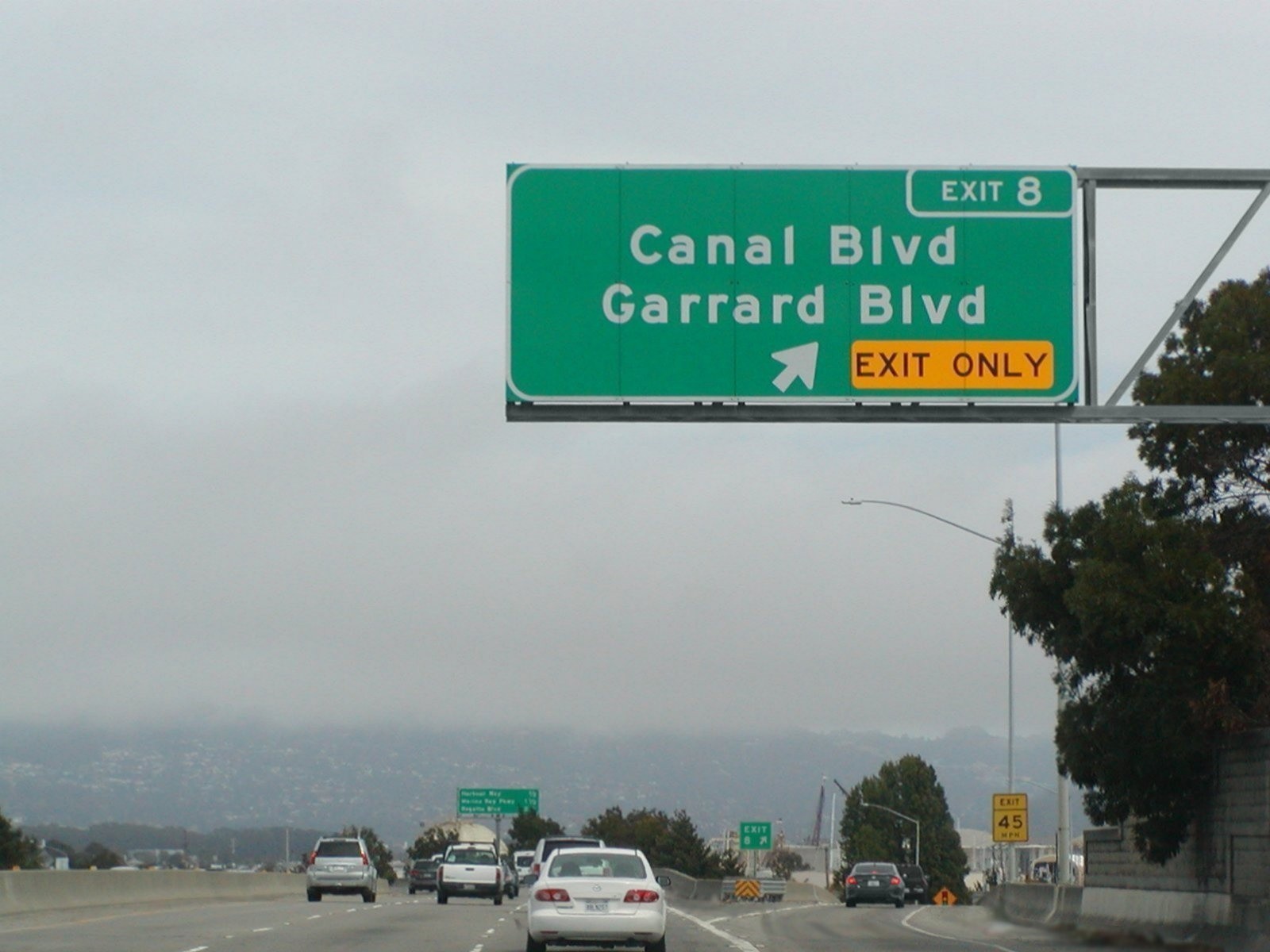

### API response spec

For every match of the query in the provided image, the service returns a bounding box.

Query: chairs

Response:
[480,855,490,864]
[614,863,637,877]
[560,863,581,876]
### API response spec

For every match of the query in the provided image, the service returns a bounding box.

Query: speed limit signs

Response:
[993,792,1030,843]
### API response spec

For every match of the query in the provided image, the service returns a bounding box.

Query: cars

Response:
[844,862,932,908]
[406,841,521,906]
[527,847,671,952]
[306,836,378,904]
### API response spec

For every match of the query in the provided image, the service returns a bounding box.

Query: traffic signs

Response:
[506,163,1087,407]
[738,821,771,850]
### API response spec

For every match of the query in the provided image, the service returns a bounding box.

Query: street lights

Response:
[859,800,921,866]
[844,496,1017,884]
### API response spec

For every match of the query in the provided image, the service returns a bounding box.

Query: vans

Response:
[512,837,616,882]
